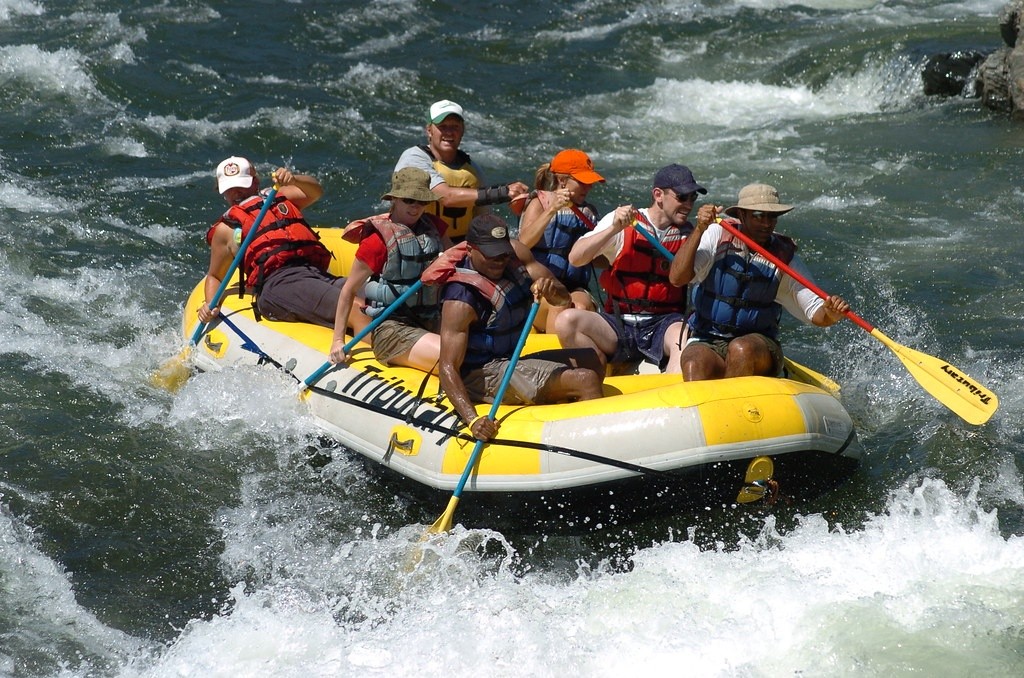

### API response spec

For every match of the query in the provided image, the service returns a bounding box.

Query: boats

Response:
[181,224,865,541]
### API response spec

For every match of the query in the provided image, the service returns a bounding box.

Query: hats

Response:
[217,156,256,195]
[466,213,514,258]
[655,164,707,195]
[724,180,794,218]
[380,167,443,201]
[426,99,465,125]
[549,149,606,185]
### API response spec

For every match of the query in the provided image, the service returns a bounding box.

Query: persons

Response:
[669,183,851,382]
[197,155,374,345]
[511,149,605,333]
[554,164,722,373]
[327,167,457,376]
[438,215,603,442]
[392,99,529,243]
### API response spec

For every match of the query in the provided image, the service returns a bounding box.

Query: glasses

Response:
[668,192,698,202]
[752,211,778,219]
[402,198,430,206]
[485,254,512,259]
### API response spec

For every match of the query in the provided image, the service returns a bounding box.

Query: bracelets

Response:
[468,416,481,430]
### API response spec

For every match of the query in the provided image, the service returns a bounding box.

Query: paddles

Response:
[629,219,841,395]
[567,201,595,230]
[298,251,444,394]
[401,293,544,571]
[148,172,281,395]
[717,215,999,426]
